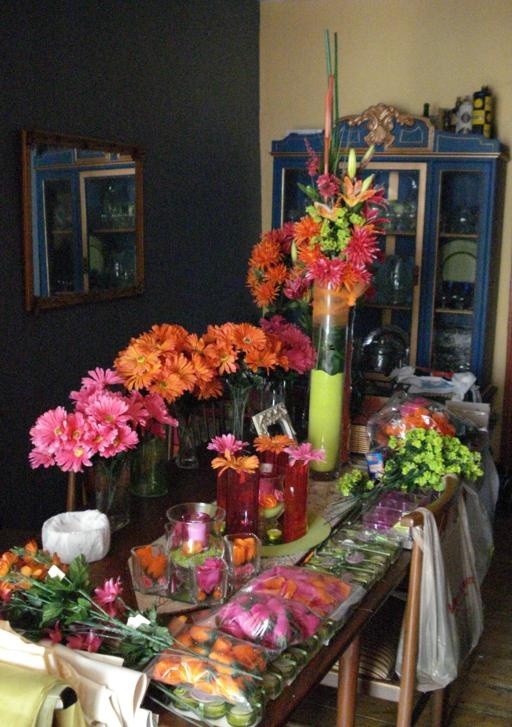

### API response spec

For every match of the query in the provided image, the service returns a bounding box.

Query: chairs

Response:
[320,478,463,724]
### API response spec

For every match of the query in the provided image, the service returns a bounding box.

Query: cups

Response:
[132,542,172,596]
[223,533,260,583]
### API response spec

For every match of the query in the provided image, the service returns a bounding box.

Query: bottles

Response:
[164,502,227,604]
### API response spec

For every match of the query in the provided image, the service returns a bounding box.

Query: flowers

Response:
[28,313,329,577]
[247,133,395,374]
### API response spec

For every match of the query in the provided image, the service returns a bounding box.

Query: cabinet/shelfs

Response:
[272,104,509,395]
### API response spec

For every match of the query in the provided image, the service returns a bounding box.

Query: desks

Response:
[91,416,438,727]
